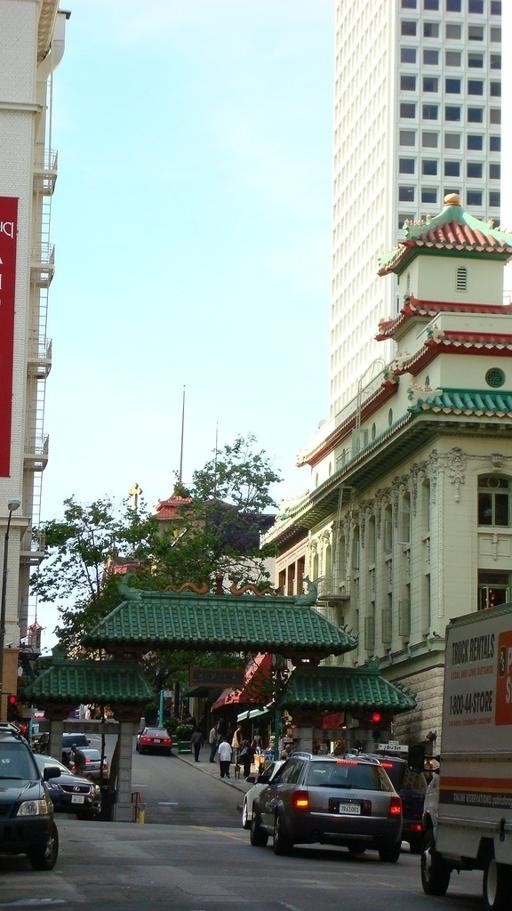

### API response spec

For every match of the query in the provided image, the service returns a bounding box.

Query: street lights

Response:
[0,498,21,692]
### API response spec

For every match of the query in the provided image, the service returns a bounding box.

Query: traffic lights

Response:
[360,709,391,730]
[8,695,18,721]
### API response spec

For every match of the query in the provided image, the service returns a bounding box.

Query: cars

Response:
[0,724,109,870]
[243,750,431,863]
[136,726,172,755]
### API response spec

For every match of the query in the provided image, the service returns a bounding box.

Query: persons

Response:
[12,719,19,726]
[84,701,113,720]
[71,746,86,776]
[190,723,257,779]
[282,744,293,759]
[19,722,28,736]
[334,739,342,753]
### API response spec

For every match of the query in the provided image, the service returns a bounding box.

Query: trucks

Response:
[421,602,512,911]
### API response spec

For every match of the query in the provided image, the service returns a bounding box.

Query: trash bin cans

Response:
[95,785,115,820]
